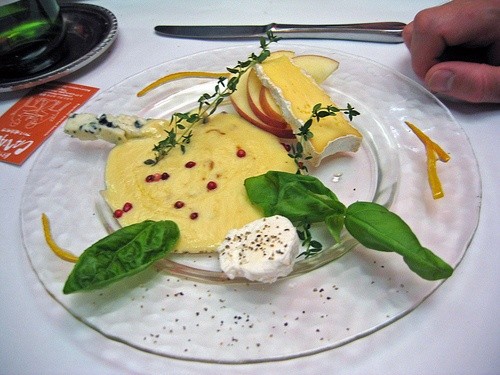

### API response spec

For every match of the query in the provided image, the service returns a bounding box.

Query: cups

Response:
[0,0,68,72]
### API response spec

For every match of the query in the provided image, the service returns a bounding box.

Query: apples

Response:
[230,50,338,135]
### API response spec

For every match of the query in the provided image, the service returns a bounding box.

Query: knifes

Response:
[154,20,408,44]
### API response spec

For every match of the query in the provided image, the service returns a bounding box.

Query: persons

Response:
[402,0,500,103]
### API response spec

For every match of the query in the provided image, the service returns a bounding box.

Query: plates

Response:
[0,2,119,94]
[17,42,484,365]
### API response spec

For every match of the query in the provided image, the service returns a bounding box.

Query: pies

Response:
[100,108,301,253]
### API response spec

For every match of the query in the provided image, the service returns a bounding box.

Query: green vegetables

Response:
[63,219,180,295]
[244,173,453,281]
